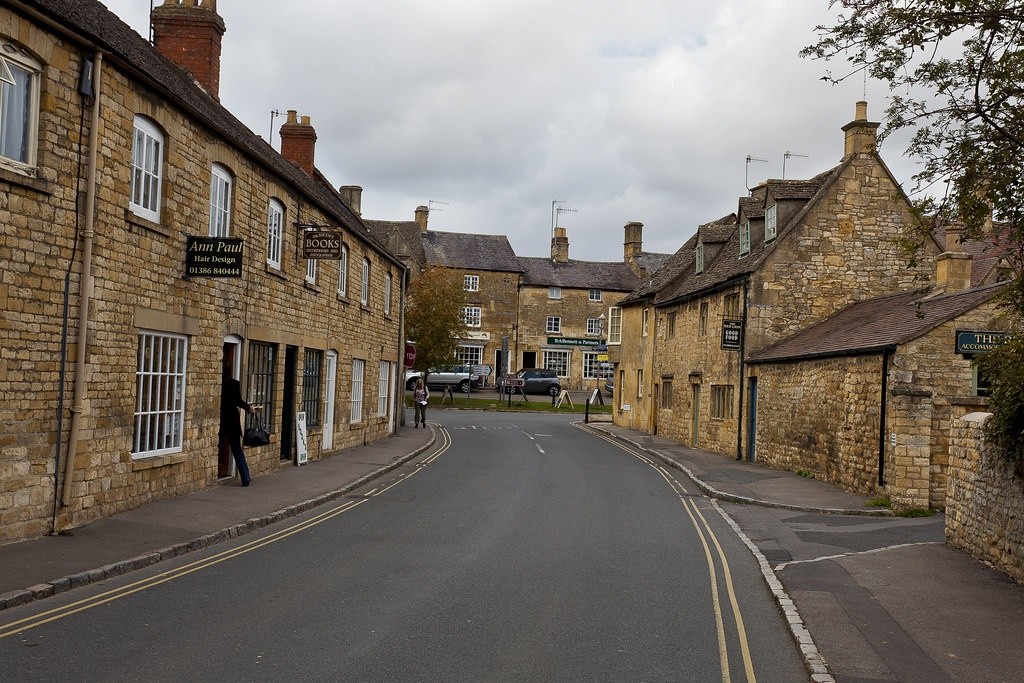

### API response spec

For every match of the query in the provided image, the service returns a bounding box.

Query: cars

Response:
[493,368,561,397]
[603,376,613,394]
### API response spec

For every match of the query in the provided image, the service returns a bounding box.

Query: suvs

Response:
[403,364,480,393]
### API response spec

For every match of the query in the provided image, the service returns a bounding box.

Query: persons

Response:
[219,361,255,486]
[414,378,431,428]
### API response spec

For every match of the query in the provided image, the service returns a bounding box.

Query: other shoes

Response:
[415,424,418,428]
[423,423,425,428]
[242,479,250,487]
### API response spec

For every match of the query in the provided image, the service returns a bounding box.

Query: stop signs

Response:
[404,345,417,369]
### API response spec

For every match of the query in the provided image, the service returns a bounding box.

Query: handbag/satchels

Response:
[243,411,270,446]
